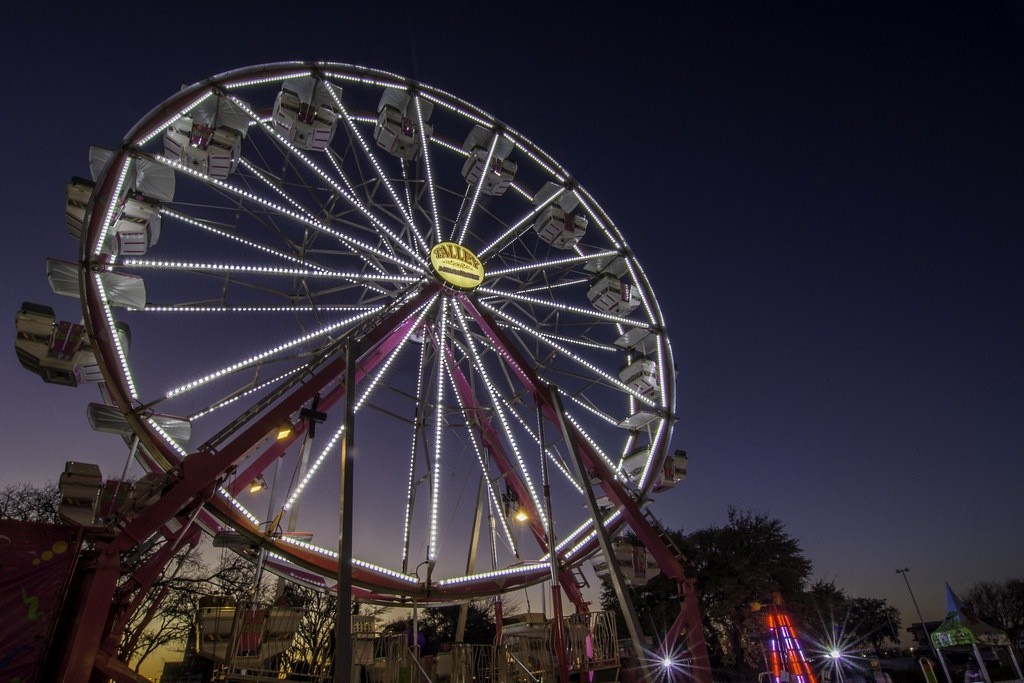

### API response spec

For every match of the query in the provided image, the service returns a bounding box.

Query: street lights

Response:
[896,567,953,683]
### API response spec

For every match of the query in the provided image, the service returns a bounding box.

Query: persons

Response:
[401,620,425,652]
[965,662,984,683]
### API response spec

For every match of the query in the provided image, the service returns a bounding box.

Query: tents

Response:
[930,581,1024,683]
